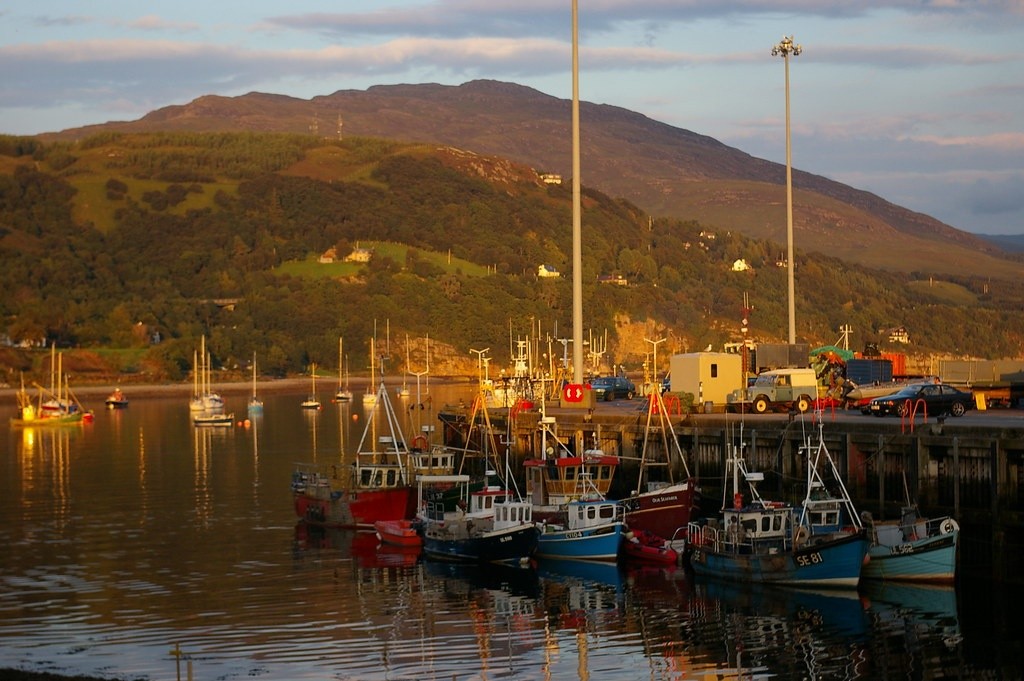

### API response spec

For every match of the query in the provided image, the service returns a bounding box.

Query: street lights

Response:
[643,337,667,383]
[771,33,804,344]
[468,348,490,391]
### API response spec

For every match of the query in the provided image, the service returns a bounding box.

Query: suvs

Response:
[725,368,818,414]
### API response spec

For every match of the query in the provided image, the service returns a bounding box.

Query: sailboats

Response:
[362,337,381,404]
[398,368,411,396]
[301,361,322,409]
[37,341,72,418]
[246,349,267,412]
[188,331,234,428]
[334,336,352,403]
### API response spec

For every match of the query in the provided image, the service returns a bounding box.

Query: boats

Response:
[104,388,130,409]
[288,359,961,592]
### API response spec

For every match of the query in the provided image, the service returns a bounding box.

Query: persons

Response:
[934,377,942,386]
[727,516,744,553]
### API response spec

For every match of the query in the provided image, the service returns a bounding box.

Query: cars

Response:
[662,378,671,396]
[589,376,636,401]
[867,382,976,417]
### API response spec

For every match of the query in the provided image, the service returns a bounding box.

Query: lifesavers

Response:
[939,518,960,535]
[411,435,429,449]
